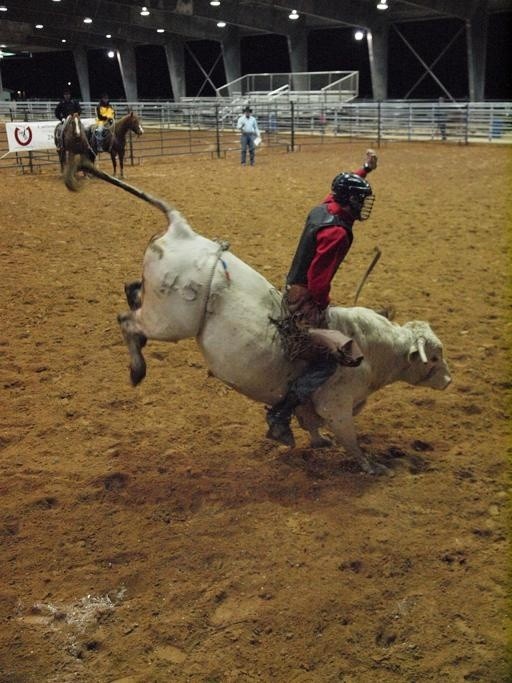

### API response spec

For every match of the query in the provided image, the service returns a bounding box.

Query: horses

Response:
[84,111,145,179]
[53,113,90,175]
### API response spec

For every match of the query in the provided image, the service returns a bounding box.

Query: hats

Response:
[242,107,252,112]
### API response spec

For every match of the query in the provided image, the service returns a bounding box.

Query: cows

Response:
[63,152,454,473]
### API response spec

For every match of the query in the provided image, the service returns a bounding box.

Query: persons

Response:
[95,93,116,152]
[237,107,260,168]
[266,149,377,449]
[434,96,448,141]
[55,88,81,124]
[73,95,79,104]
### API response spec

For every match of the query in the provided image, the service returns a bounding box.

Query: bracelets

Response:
[364,163,372,172]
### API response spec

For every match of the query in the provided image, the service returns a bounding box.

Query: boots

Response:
[266,390,300,448]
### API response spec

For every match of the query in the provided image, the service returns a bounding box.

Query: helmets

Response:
[332,172,372,209]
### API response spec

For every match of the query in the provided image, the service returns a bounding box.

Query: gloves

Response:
[364,149,377,171]
